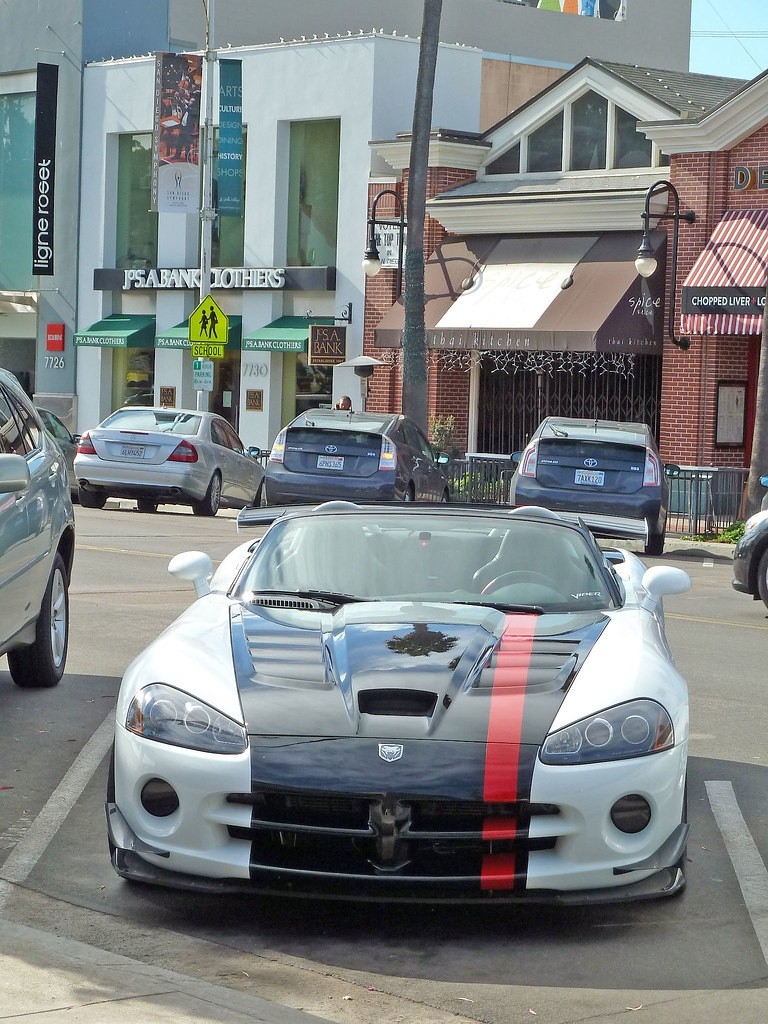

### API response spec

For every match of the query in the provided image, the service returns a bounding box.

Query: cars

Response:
[509,415,681,557]
[732,508,768,608]
[0,368,76,690]
[35,405,83,503]
[264,410,452,504]
[72,407,266,518]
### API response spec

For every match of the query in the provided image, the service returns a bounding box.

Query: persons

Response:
[331,395,352,411]
[159,53,200,160]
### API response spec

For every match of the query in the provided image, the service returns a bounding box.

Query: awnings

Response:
[242,316,335,353]
[154,315,242,351]
[73,314,155,349]
[374,230,667,379]
[679,210,768,335]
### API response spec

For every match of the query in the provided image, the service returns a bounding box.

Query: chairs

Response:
[472,506,588,598]
[275,502,386,595]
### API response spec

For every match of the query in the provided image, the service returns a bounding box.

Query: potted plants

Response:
[300,163,312,233]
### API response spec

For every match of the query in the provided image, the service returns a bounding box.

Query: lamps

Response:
[333,355,393,378]
[635,180,695,351]
[362,189,407,298]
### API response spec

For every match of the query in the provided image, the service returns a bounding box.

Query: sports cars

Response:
[105,500,691,908]
